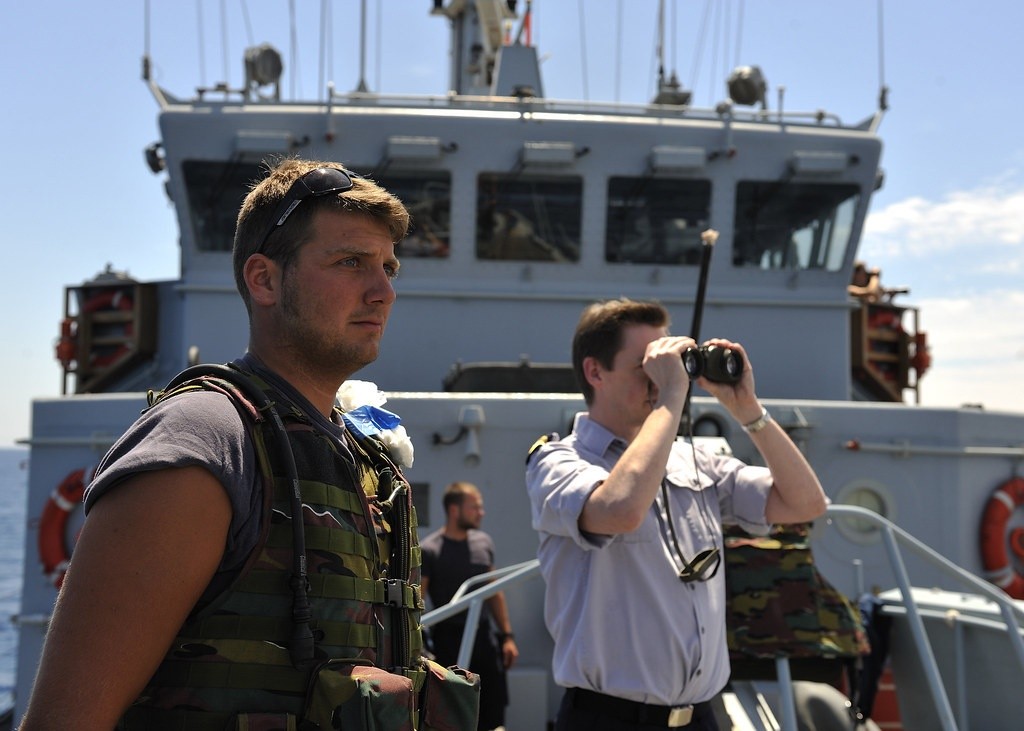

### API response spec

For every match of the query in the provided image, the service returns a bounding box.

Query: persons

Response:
[15,160,424,730]
[526,299,828,731]
[418,481,519,731]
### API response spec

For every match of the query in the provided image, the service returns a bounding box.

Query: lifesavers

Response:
[39,470,91,588]
[79,294,133,367]
[980,477,1024,600]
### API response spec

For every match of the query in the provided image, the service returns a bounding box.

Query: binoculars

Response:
[681,343,744,384]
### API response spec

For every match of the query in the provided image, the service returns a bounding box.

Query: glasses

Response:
[257,167,363,253]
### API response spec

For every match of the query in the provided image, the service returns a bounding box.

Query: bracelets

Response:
[741,407,772,434]
[504,632,515,639]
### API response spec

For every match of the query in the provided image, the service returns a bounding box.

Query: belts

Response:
[567,687,712,727]
